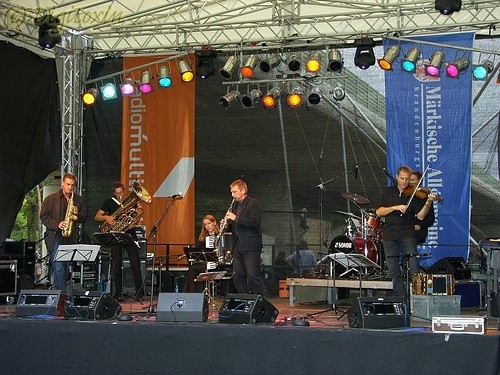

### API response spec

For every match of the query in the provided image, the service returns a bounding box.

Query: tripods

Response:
[93,231,142,302]
[300,168,356,321]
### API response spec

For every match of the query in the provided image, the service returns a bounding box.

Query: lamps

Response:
[354,37,378,70]
[473,51,495,81]
[423,45,448,76]
[259,49,283,73]
[196,50,218,80]
[218,79,346,112]
[33,14,62,50]
[305,45,323,73]
[240,50,258,80]
[101,77,118,101]
[378,38,401,71]
[326,44,344,74]
[176,56,195,85]
[83,81,100,106]
[219,51,241,80]
[447,51,471,78]
[286,48,302,72]
[119,72,139,97]
[435,0,463,16]
[155,61,175,88]
[403,41,423,73]
[139,67,156,95]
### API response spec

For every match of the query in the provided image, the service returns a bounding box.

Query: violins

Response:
[403,182,444,203]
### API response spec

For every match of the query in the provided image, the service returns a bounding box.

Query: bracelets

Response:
[424,204,430,208]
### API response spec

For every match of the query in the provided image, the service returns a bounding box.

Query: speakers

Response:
[156,292,209,321]
[64,290,122,321]
[15,290,68,318]
[219,293,279,324]
[485,247,500,321]
[426,256,471,281]
[0,241,36,293]
[346,295,410,329]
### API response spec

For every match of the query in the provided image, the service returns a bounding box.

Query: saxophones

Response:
[62,191,78,237]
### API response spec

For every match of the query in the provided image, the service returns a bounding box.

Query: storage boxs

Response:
[0,260,18,304]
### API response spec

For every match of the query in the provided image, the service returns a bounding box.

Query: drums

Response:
[326,234,379,279]
[357,212,383,237]
[379,230,384,241]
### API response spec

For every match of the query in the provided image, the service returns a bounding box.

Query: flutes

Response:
[213,198,237,252]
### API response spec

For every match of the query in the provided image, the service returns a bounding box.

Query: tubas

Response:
[101,180,152,234]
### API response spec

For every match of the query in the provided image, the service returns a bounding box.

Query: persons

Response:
[40,174,88,292]
[183,214,219,293]
[409,172,435,245]
[289,240,317,278]
[376,166,436,301]
[219,180,267,298]
[94,181,144,301]
[275,250,286,278]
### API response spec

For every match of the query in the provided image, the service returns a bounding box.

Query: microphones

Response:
[169,192,183,198]
[355,164,358,179]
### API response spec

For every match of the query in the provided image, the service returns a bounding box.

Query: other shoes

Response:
[114,294,120,299]
[137,295,144,303]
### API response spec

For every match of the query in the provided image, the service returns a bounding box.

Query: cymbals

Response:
[340,193,370,204]
[335,210,362,220]
